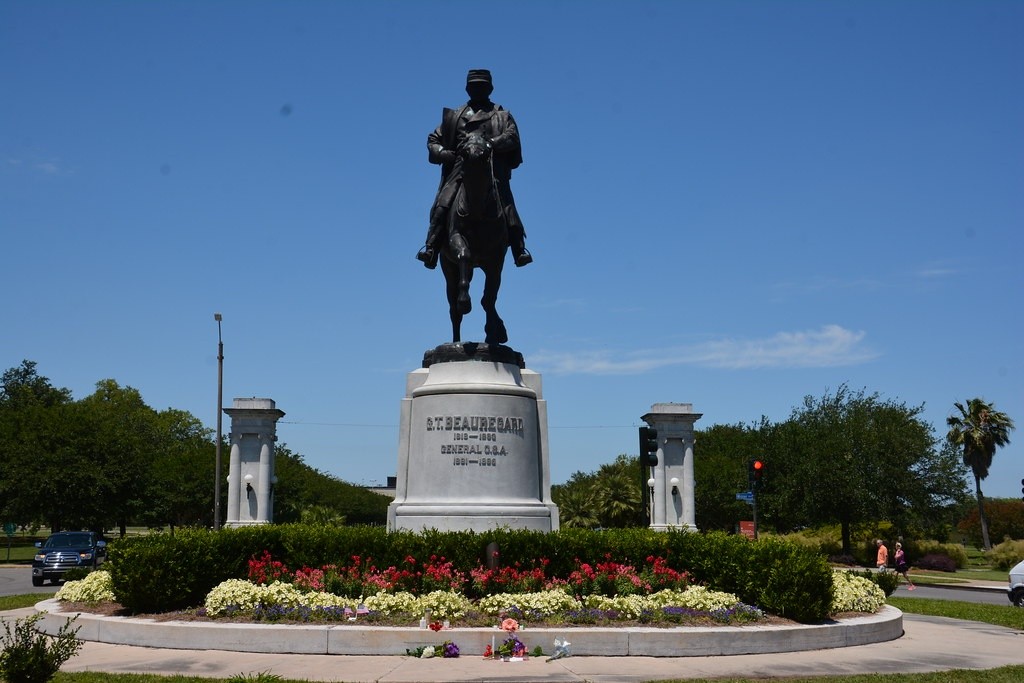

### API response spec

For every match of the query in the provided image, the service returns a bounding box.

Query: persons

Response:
[894,543,916,591]
[414,69,534,269]
[876,539,887,575]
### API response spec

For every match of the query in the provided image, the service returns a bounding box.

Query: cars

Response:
[1007,558,1024,609]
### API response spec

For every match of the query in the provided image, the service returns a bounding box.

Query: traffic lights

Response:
[640,425,659,467]
[748,459,765,492]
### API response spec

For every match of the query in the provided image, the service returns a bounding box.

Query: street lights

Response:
[213,312,223,532]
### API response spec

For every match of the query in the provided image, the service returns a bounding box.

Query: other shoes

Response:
[908,585,916,591]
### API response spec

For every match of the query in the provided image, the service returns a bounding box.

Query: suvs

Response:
[30,532,108,587]
